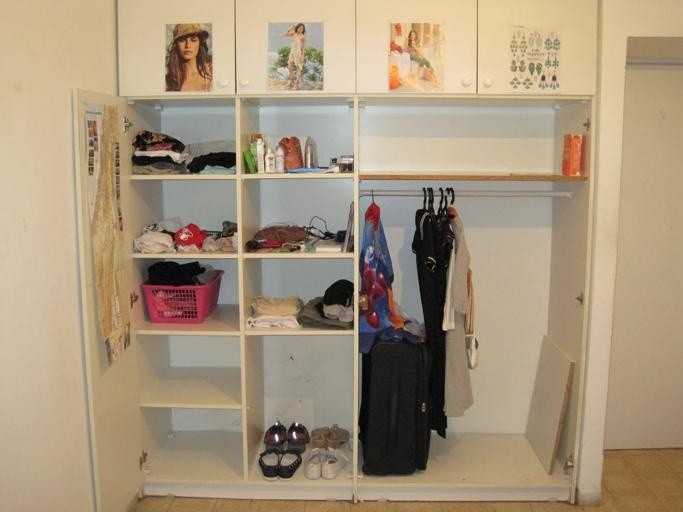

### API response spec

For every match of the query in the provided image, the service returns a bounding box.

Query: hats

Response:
[170,23,210,45]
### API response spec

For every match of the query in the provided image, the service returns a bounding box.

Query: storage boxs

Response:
[139,269,227,324]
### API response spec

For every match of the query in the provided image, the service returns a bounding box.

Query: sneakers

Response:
[264,420,287,450]
[278,449,302,480]
[287,422,311,453]
[304,448,328,479]
[321,447,350,480]
[258,449,280,481]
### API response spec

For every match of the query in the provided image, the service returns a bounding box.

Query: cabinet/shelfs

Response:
[114,2,357,96]
[0,0,117,512]
[353,2,601,95]
[68,94,599,503]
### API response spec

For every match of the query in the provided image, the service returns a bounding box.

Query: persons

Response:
[165,23,213,92]
[404,30,438,83]
[282,23,306,89]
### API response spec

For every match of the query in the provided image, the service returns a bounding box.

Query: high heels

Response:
[311,425,330,448]
[327,424,350,449]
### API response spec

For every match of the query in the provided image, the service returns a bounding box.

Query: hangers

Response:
[442,188,450,220]
[369,189,378,212]
[428,187,436,217]
[449,188,456,214]
[421,185,427,213]
[438,187,443,219]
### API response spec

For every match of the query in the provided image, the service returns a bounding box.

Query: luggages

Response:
[359,331,433,476]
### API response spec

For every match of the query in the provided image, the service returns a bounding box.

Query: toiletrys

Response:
[329,158,341,173]
[305,136,314,169]
[265,148,275,174]
[276,145,284,174]
